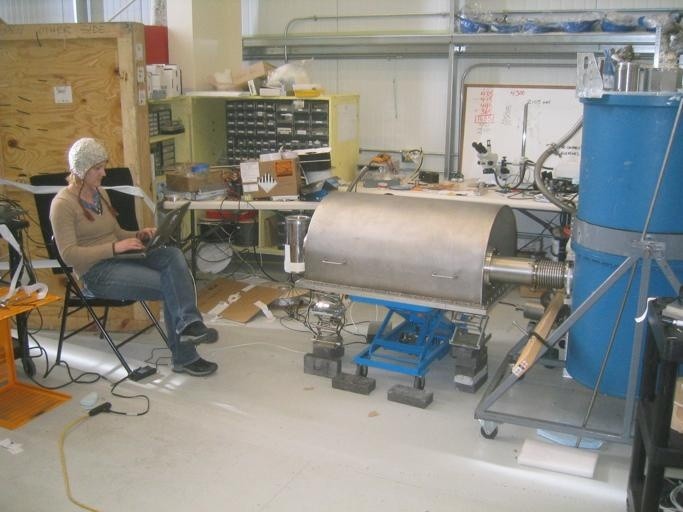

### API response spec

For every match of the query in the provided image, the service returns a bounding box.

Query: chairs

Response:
[51,235,172,374]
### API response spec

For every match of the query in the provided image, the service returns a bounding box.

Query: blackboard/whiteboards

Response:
[457,84,584,185]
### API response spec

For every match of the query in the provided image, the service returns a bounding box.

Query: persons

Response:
[49,137,220,377]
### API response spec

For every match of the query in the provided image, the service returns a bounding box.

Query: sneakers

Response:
[179,320,218,344]
[172,357,218,376]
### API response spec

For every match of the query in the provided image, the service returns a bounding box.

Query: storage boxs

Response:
[231,61,276,91]
[240,157,301,200]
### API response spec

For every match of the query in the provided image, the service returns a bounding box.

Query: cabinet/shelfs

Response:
[225,100,328,164]
[627,297,683,512]
[149,95,192,181]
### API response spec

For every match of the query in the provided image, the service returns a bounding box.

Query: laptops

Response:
[109,200,192,262]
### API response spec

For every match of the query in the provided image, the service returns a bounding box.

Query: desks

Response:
[0,287,71,430]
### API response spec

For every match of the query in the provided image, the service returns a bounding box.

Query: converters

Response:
[129,364,156,382]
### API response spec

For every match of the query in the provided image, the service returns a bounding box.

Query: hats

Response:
[68,138,120,221]
